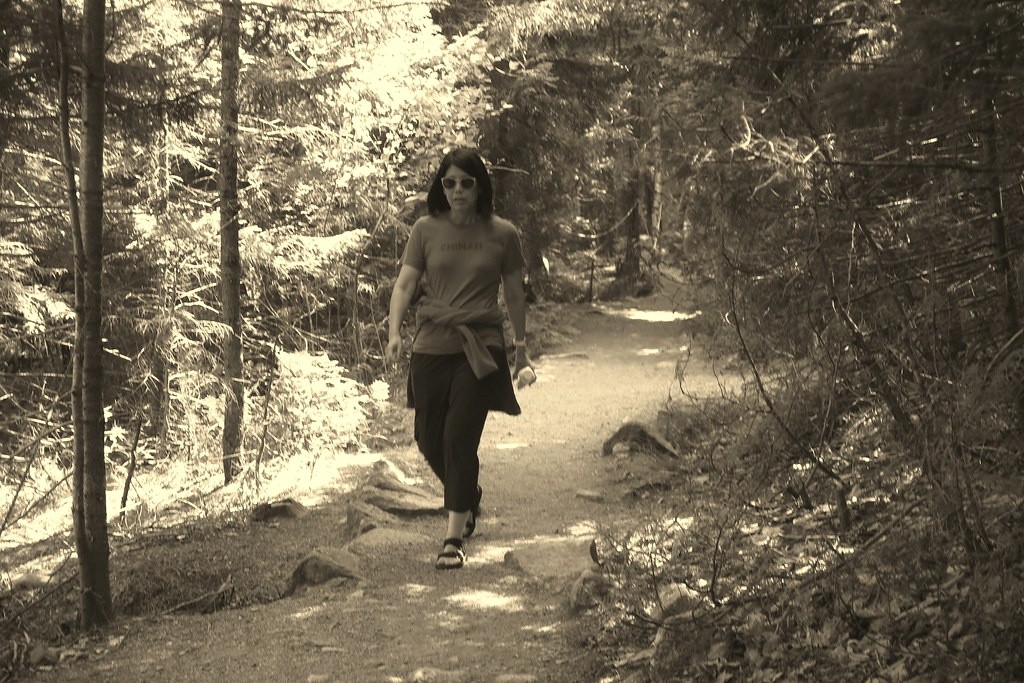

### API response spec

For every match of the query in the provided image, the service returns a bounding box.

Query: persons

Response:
[385,149,536,570]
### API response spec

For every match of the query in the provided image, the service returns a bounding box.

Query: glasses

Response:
[441,176,476,191]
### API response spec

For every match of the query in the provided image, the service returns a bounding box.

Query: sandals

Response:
[463,485,482,538]
[435,538,467,570]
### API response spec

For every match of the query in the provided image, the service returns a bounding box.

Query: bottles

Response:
[518,366,535,385]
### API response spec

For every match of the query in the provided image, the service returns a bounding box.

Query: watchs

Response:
[513,336,526,347]
